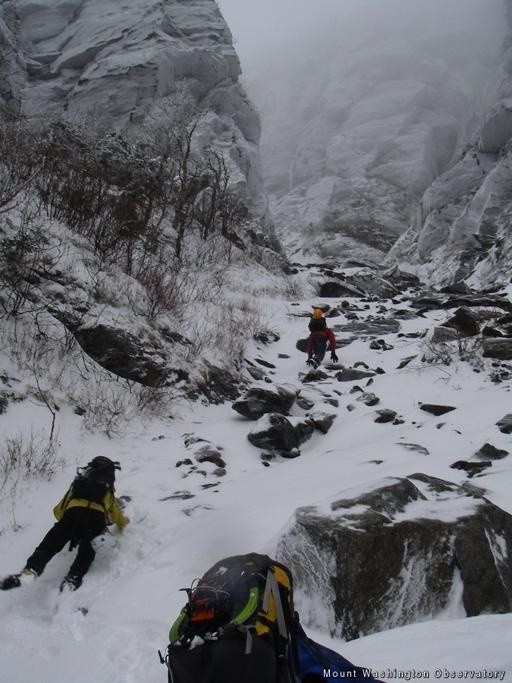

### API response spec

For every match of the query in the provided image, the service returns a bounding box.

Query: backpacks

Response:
[71,456,121,504]
[179,549,295,644]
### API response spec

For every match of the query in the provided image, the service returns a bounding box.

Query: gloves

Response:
[330,352,338,363]
[305,356,312,366]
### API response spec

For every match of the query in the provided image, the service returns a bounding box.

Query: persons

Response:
[305,328,338,368]
[0,456,129,591]
[167,553,384,683]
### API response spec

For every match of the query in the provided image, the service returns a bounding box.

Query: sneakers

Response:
[310,358,320,369]
[59,574,81,592]
[0,572,34,591]
